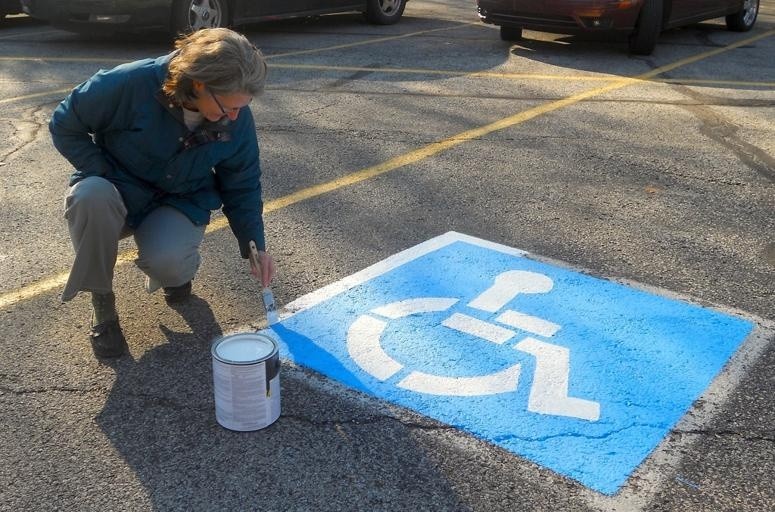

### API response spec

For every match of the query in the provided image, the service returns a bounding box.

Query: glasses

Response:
[208,87,241,116]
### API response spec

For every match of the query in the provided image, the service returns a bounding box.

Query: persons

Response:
[47,25,277,360]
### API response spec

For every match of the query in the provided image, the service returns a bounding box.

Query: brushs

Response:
[249,241,278,325]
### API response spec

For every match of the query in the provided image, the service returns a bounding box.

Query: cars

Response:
[476,0,761,55]
[0,0,408,41]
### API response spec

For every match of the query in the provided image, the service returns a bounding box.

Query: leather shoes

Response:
[164,280,192,303]
[88,307,127,357]
